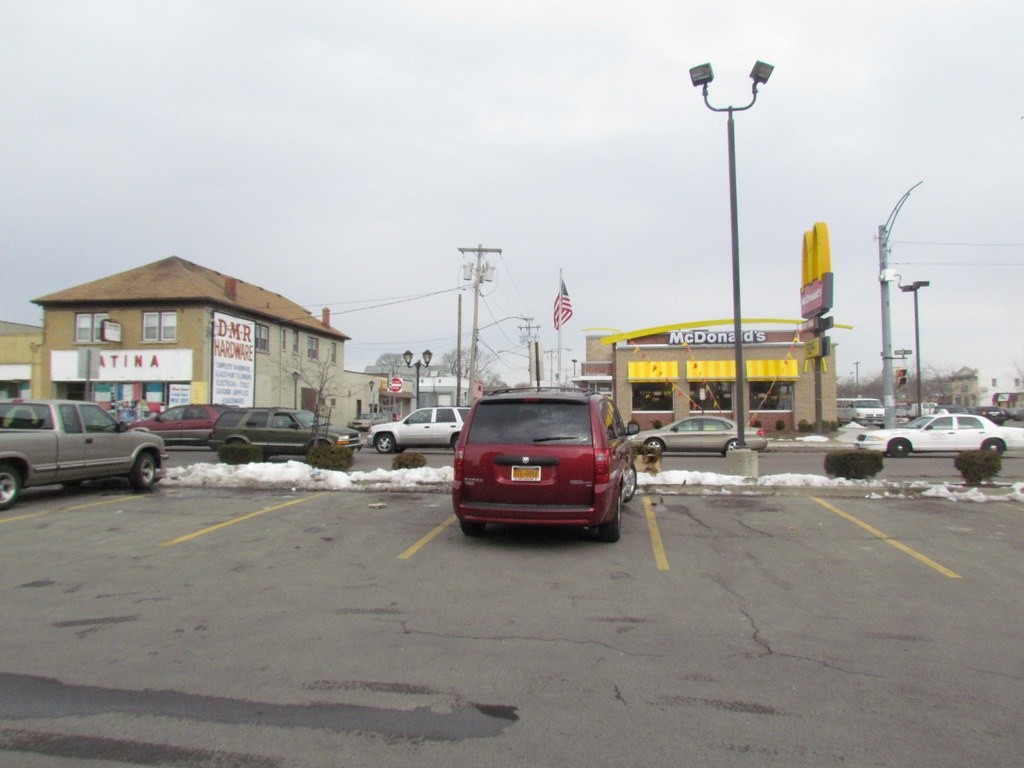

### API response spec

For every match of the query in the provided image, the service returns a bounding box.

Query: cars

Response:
[627,416,770,454]
[910,401,1024,429]
[854,413,1024,461]
[128,404,243,451]
[347,412,386,431]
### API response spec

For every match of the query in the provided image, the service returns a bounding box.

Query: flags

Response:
[553,279,574,331]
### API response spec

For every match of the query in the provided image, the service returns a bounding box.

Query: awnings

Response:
[627,360,800,382]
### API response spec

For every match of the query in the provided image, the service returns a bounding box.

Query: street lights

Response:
[902,281,930,416]
[403,349,433,409]
[496,347,572,387]
[686,60,774,449]
[466,316,536,407]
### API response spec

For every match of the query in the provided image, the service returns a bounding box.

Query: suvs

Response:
[449,385,638,544]
[0,397,171,512]
[367,405,473,453]
[206,406,364,465]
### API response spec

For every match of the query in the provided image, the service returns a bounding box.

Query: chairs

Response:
[509,410,546,439]
[690,421,698,431]
[187,412,194,419]
[419,413,431,423]
[195,410,203,419]
[8,410,36,428]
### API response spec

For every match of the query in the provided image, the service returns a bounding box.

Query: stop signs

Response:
[389,378,403,393]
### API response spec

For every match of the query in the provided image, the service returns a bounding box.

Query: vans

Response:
[836,397,886,430]
